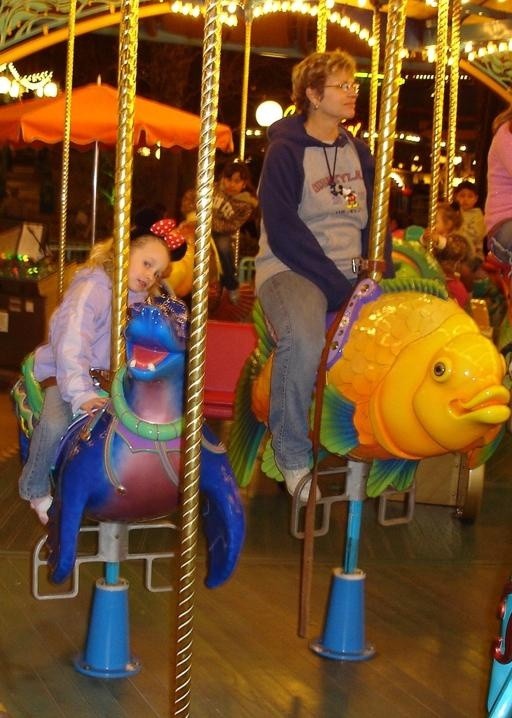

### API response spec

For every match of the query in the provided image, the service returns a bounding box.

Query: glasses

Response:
[323,81,358,92]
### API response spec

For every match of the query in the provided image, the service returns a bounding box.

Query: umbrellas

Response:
[0,76,234,249]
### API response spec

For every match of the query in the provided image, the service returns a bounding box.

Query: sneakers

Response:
[30,493,54,523]
[274,455,320,506]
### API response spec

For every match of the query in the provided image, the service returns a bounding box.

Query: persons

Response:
[455,181,485,292]
[485,103,512,267]
[254,51,390,503]
[419,205,471,276]
[17,233,174,526]
[213,163,249,305]
